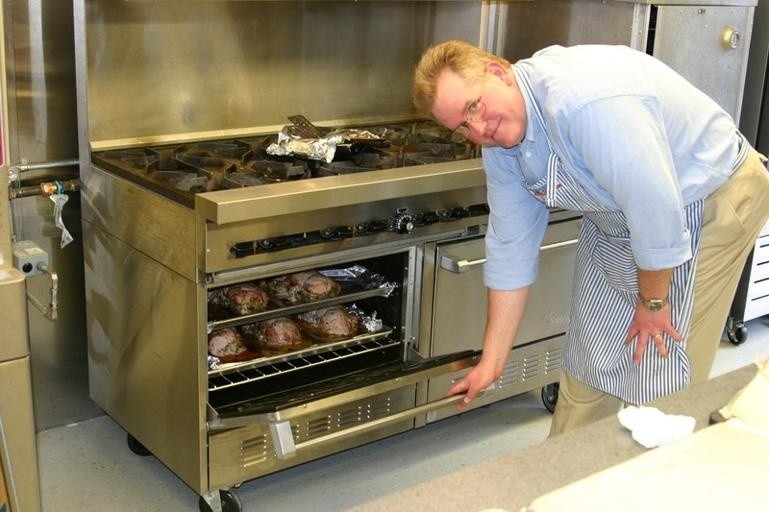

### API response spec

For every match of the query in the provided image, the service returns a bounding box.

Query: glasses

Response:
[444,60,490,144]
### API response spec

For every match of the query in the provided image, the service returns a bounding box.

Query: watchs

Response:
[637,290,670,312]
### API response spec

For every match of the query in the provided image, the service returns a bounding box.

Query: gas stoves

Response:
[89,115,489,209]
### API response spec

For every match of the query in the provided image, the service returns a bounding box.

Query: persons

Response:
[409,40,769,438]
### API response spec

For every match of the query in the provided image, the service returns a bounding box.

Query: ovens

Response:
[199,206,596,423]
